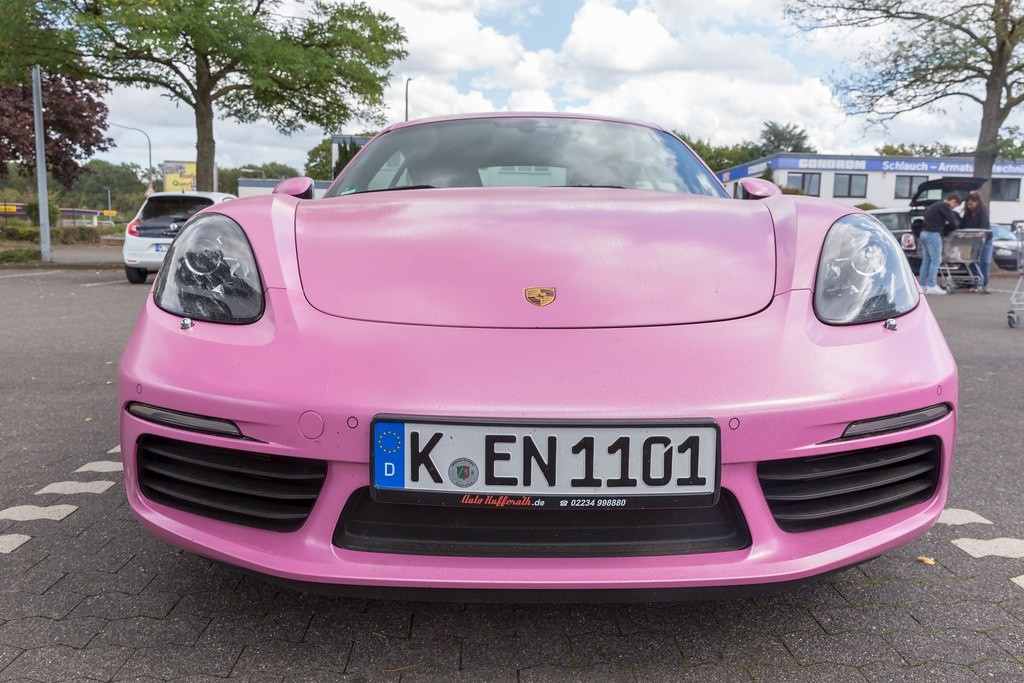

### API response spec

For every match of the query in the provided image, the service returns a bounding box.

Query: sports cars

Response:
[114,111,961,606]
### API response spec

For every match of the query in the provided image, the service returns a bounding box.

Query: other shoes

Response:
[920,285,927,294]
[925,285,947,295]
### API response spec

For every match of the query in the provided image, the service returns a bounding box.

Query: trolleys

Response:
[1005,218,1024,329]
[913,226,994,297]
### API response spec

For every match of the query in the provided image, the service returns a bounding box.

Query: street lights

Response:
[112,124,154,194]
[406,76,412,122]
[241,168,266,179]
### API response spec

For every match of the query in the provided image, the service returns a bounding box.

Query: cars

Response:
[859,175,994,278]
[121,190,241,283]
[988,223,1022,271]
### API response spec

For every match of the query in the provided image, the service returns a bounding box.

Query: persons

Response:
[918,194,962,295]
[964,191,993,291]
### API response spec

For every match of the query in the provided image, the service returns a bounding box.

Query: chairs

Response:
[569,160,637,188]
[407,153,483,187]
